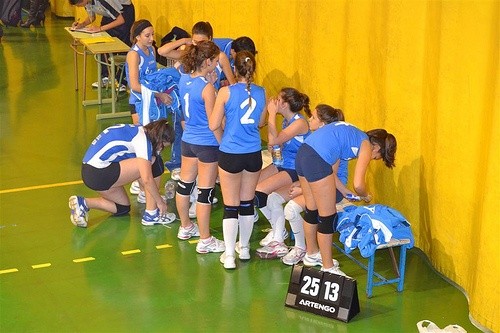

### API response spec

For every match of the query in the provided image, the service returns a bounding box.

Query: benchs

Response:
[261,148,409,299]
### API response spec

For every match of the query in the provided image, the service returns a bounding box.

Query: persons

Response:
[20,0,47,29]
[125,19,397,279]
[68,0,135,93]
[68,118,176,227]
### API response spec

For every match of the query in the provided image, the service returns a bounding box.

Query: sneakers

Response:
[234,241,250,259]
[321,266,346,277]
[141,209,176,225]
[69,196,89,228]
[171,168,180,180]
[129,182,139,195]
[259,228,289,247]
[256,241,289,257]
[137,192,167,203]
[302,253,340,267]
[189,187,218,204]
[189,201,197,218]
[196,236,225,253]
[177,221,200,239]
[116,83,126,92]
[219,252,236,269]
[280,248,306,265]
[92,77,108,88]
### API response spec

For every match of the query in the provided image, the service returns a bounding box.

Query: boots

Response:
[21,0,41,28]
[37,0,46,26]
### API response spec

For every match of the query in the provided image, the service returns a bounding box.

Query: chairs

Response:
[104,40,136,99]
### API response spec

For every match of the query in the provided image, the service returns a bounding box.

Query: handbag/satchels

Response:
[417,319,467,333]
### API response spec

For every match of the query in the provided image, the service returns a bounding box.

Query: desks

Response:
[82,36,137,121]
[64,25,111,98]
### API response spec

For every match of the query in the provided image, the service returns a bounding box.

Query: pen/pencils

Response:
[74,18,80,26]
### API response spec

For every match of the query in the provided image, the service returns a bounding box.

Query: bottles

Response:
[164,179,175,199]
[272,145,284,166]
